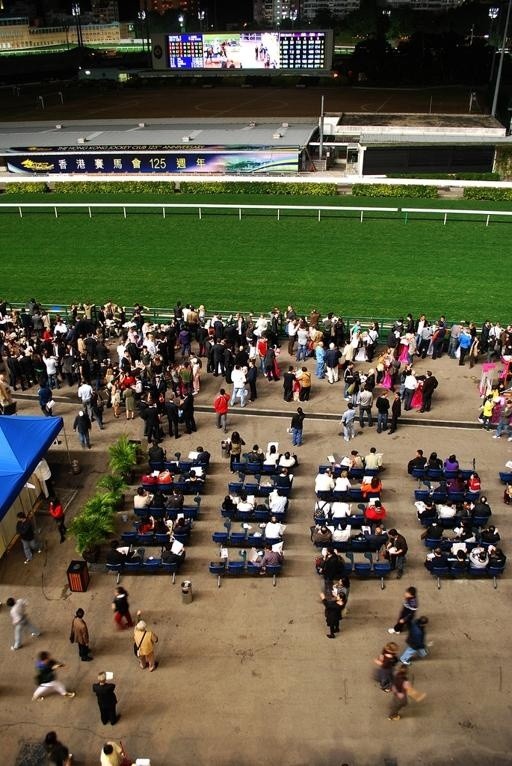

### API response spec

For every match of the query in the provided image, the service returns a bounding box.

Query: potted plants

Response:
[64,436,150,564]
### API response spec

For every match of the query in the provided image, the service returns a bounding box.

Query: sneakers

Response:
[32,632,40,636]
[388,715,400,721]
[32,696,44,702]
[64,692,75,699]
[24,559,30,564]
[60,537,65,543]
[149,665,155,672]
[64,530,66,534]
[381,687,390,693]
[11,644,23,651]
[37,547,41,553]
[400,659,412,665]
[388,628,400,635]
[140,662,146,669]
[327,634,335,638]
[334,630,339,633]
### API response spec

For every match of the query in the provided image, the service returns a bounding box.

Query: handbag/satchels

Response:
[69,632,75,643]
[287,427,294,435]
[134,643,139,656]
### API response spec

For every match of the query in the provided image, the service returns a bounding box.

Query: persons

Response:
[132,620,160,671]
[318,590,348,638]
[42,729,72,766]
[0,293,512,577]
[91,671,122,726]
[4,594,42,651]
[399,615,430,665]
[100,739,126,766]
[374,640,405,692]
[68,604,95,662]
[388,586,419,634]
[111,584,133,631]
[30,651,76,703]
[205,37,279,68]
[319,545,341,592]
[331,576,353,614]
[387,663,427,721]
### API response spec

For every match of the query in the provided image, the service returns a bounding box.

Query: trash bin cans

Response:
[181,581,192,604]
[67,560,90,592]
[221,440,229,458]
[72,460,80,474]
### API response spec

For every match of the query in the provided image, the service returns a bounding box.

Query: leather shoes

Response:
[111,714,121,725]
[81,656,93,661]
[103,720,108,725]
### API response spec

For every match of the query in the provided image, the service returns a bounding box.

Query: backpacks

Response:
[333,556,344,580]
